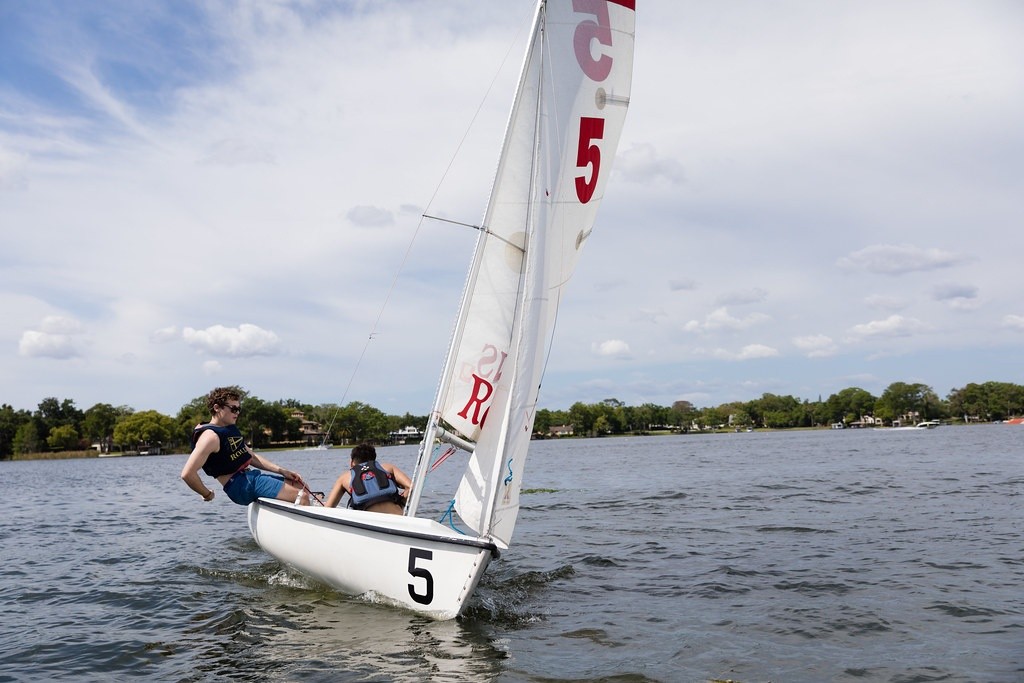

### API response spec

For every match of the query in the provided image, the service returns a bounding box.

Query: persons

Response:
[180,388,312,506]
[323,445,415,516]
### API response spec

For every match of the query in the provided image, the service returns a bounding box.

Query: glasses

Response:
[224,404,241,414]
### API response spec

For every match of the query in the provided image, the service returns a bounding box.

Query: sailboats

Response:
[247,1,638,620]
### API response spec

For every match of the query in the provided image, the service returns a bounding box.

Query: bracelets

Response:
[203,490,212,498]
